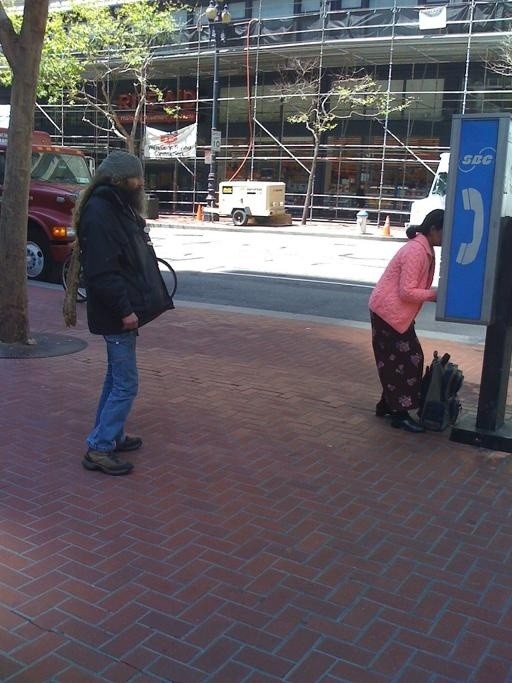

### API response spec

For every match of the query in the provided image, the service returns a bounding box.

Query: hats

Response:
[99,149,144,178]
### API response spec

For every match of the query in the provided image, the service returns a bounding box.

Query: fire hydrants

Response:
[356,211,369,234]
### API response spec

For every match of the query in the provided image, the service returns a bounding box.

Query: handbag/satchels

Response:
[422,350,464,432]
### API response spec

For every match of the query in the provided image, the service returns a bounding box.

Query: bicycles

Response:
[61,247,178,304]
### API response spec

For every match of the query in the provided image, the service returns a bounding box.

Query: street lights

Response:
[205,0,232,206]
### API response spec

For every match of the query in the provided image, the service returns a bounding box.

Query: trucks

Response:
[0,127,92,281]
[404,153,450,228]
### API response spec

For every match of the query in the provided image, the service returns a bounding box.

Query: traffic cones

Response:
[381,216,392,237]
[197,204,204,220]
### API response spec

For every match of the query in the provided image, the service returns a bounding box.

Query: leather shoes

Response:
[375,402,425,433]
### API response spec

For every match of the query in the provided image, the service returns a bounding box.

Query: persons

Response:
[70,149,175,476]
[367,209,445,434]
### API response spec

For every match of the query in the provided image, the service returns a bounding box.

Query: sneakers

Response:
[81,434,143,476]
